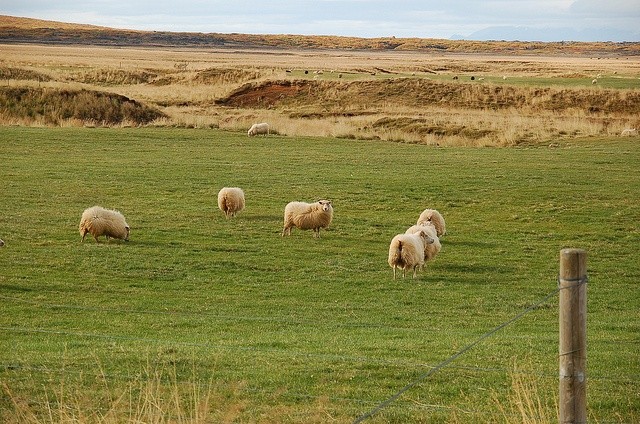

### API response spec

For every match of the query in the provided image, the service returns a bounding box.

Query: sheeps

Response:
[404,220,442,261]
[419,206,448,241]
[78,208,129,245]
[218,185,245,217]
[281,198,336,236]
[388,231,434,278]
[247,122,270,137]
